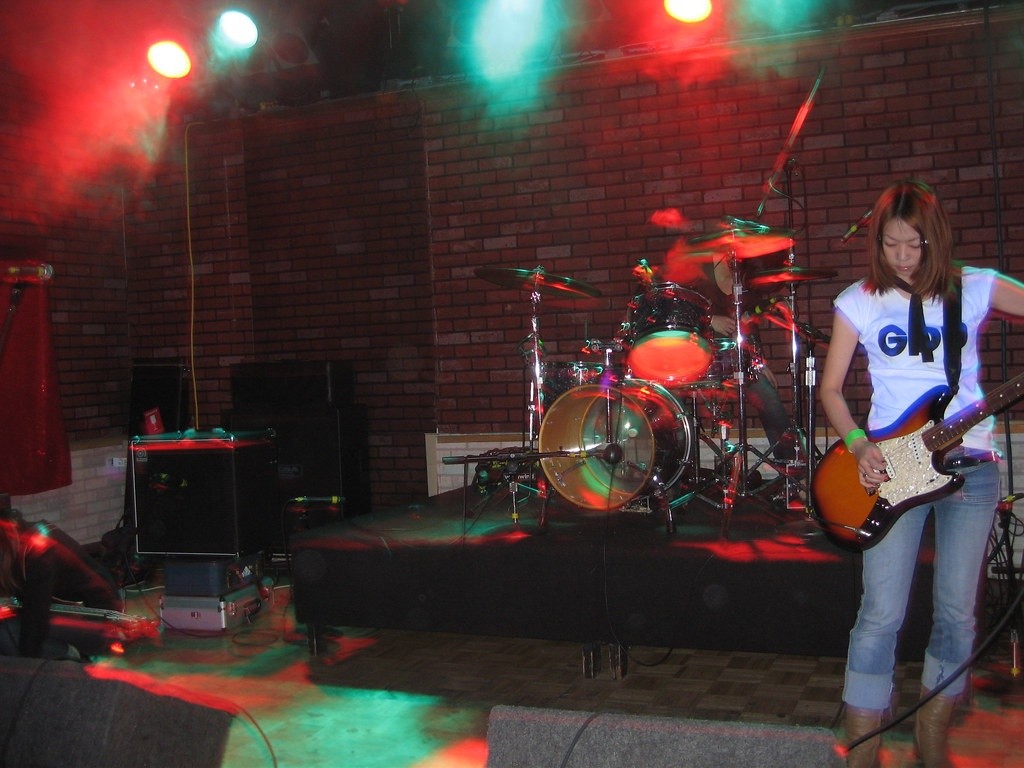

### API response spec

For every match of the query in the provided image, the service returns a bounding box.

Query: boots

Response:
[914,683,957,768]
[846,704,881,768]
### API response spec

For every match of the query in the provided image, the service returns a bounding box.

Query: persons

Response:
[819,175,1024,768]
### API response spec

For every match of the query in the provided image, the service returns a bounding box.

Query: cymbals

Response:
[673,235,798,257]
[746,271,839,287]
[473,265,605,302]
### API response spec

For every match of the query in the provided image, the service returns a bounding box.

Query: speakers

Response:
[130,429,288,561]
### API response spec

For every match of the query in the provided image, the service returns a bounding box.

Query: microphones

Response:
[741,296,782,322]
[724,215,766,228]
[601,443,622,465]
[840,210,873,244]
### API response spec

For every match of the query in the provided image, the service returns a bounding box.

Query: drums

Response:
[537,379,690,511]
[535,361,633,417]
[707,338,758,388]
[624,285,713,387]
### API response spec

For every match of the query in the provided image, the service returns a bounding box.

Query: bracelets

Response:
[844,429,868,453]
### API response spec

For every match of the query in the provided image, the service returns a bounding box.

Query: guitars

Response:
[809,371,1024,553]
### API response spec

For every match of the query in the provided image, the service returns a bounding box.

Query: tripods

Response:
[645,65,828,541]
